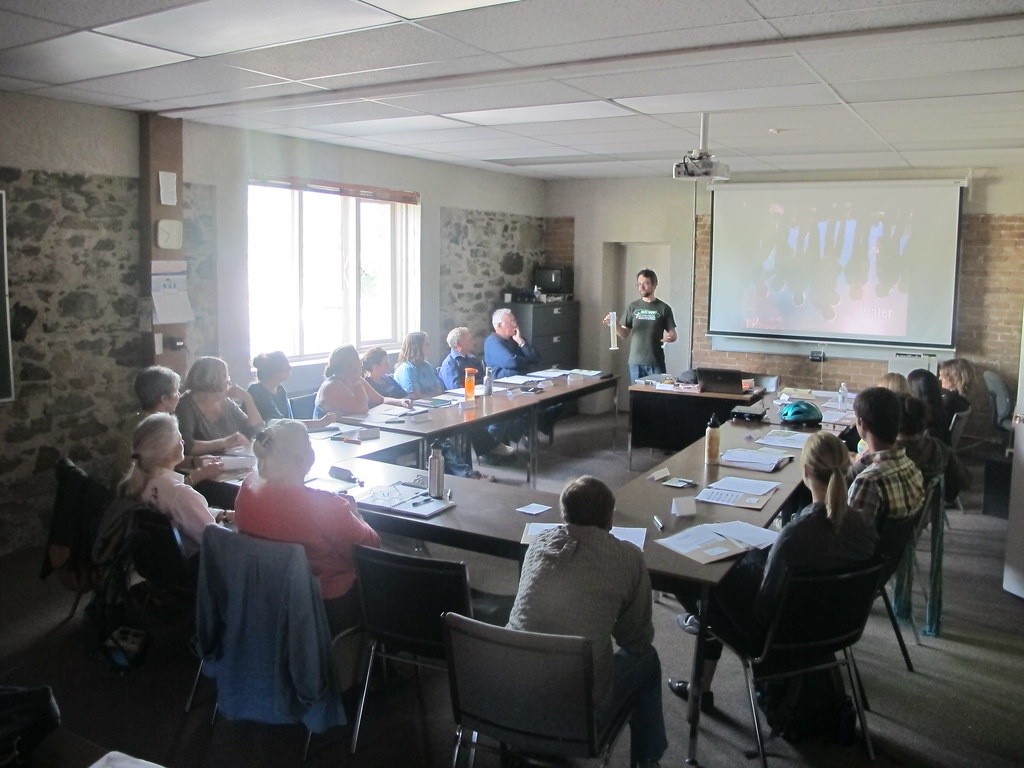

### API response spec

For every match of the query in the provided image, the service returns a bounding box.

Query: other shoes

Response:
[773,516,782,532]
[668,678,714,710]
[470,471,496,482]
[678,612,699,635]
[489,442,513,456]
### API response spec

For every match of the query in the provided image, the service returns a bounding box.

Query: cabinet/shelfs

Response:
[497,301,578,420]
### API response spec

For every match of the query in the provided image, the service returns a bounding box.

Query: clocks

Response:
[155,218,182,249]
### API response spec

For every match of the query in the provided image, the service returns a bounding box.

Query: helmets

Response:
[780,400,823,424]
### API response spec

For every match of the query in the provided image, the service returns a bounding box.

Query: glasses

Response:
[369,485,402,498]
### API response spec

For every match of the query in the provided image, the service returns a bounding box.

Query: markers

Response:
[343,438,360,444]
[331,437,345,441]
[549,380,555,386]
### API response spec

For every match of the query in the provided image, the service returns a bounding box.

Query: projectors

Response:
[731,405,766,419]
[673,160,730,180]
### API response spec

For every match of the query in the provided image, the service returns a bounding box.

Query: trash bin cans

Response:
[980,459,1011,518]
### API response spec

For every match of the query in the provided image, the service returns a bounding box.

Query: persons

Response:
[603,269,678,385]
[233,418,382,622]
[505,474,668,768]
[668,431,879,711]
[116,412,238,568]
[115,309,979,768]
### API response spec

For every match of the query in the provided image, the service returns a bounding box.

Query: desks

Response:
[178,374,857,764]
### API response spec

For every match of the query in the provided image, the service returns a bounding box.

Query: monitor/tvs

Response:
[534,266,573,294]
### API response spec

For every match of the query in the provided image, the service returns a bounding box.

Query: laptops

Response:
[695,367,750,395]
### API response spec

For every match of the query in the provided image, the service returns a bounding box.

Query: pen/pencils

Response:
[318,405,327,414]
[398,412,407,417]
[654,516,664,528]
[412,498,431,505]
[385,420,405,424]
[447,489,451,500]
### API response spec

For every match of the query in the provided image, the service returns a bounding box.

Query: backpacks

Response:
[752,653,846,743]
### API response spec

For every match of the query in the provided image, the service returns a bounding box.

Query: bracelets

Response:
[223,509,229,524]
[186,474,195,485]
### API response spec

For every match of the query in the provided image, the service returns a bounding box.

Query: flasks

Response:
[428,439,444,499]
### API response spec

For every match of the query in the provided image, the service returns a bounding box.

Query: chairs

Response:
[984,370,1016,457]
[724,405,972,768]
[288,391,316,424]
[41,457,643,768]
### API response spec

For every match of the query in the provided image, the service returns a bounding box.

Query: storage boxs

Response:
[577,383,616,414]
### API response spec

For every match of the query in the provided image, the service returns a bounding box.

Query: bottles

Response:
[465,368,478,400]
[838,382,848,410]
[705,412,720,465]
[483,367,493,397]
